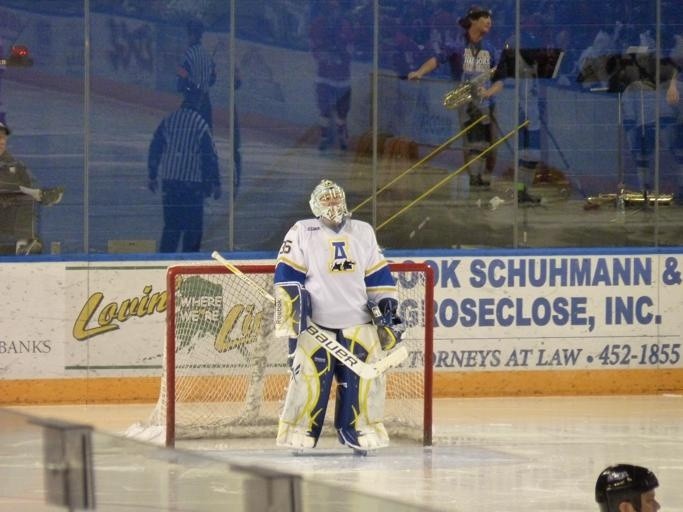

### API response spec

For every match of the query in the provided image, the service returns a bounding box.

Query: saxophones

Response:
[442,40,510,111]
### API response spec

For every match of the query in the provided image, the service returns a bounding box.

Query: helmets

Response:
[0,112,10,135]
[465,5,492,17]
[309,180,352,223]
[594,463,659,502]
[185,16,205,35]
[183,83,203,107]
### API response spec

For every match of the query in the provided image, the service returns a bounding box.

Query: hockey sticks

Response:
[211,250,408,381]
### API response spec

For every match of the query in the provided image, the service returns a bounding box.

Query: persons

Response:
[119,0,683,94]
[313,34,350,155]
[147,15,221,256]
[1,110,65,256]
[608,59,678,194]
[271,177,407,459]
[407,7,508,186]
[596,462,662,512]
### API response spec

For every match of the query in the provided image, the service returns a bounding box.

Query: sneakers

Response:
[469,175,482,185]
[517,192,541,203]
